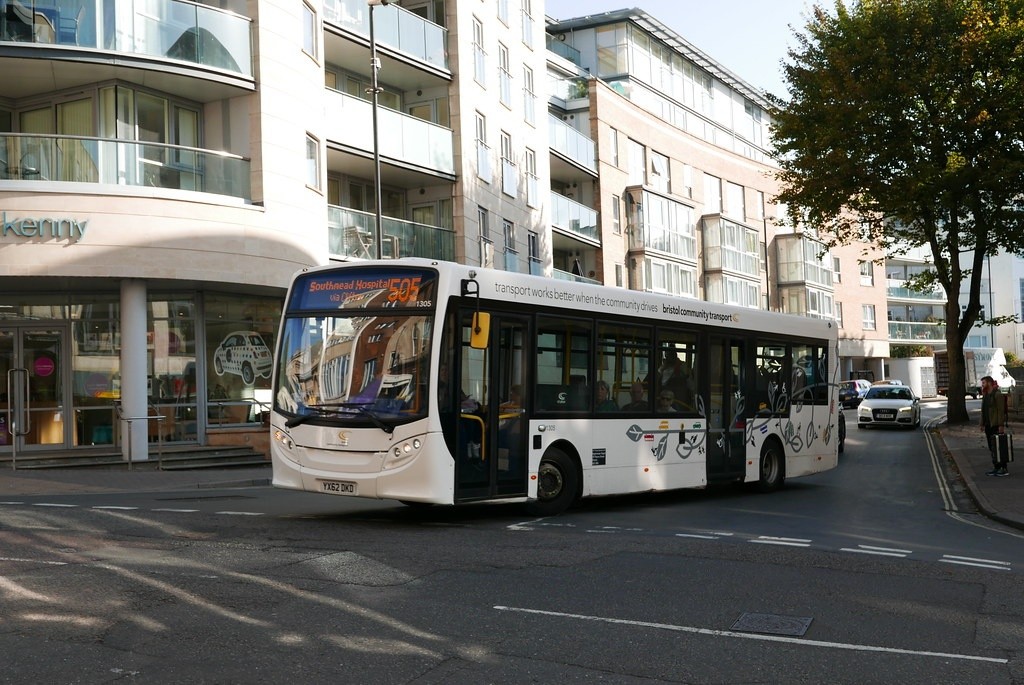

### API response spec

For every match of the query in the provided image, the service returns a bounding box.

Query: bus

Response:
[269,256,842,522]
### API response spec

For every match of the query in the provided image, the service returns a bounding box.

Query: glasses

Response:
[660,396,672,401]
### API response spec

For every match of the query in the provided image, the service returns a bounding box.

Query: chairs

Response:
[728,364,805,409]
[398,236,417,255]
[59,5,87,48]
[342,225,372,261]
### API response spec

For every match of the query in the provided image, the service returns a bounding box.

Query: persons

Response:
[657,343,695,390]
[498,385,526,414]
[621,382,648,411]
[978,376,1010,477]
[424,364,482,459]
[775,354,795,375]
[588,381,618,412]
[657,390,678,411]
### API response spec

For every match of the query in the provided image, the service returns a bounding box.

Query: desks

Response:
[371,234,399,259]
[4,4,59,42]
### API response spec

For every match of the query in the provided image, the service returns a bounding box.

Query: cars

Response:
[857,380,922,430]
[837,379,871,408]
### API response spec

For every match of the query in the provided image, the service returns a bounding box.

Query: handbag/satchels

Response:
[991,433,1014,465]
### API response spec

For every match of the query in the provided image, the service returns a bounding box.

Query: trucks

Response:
[933,347,1016,400]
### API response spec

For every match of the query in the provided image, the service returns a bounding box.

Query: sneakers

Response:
[995,469,1010,477]
[985,469,997,476]
